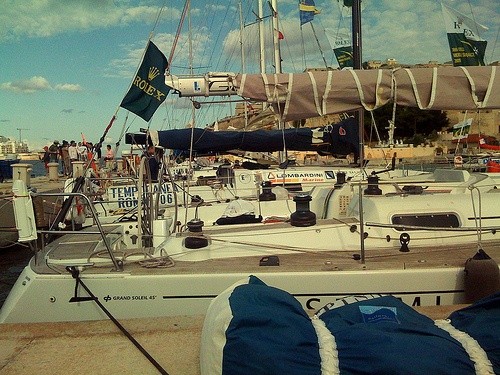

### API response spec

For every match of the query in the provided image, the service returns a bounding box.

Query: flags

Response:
[118,40,174,122]
[444,118,472,140]
[441,3,488,67]
[324,28,354,70]
[299,0,320,26]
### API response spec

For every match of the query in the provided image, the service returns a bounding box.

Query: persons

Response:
[141,145,156,159]
[41,146,49,174]
[47,140,60,163]
[58,140,99,179]
[103,145,114,162]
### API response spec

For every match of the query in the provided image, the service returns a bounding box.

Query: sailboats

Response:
[0,0,500,324]
